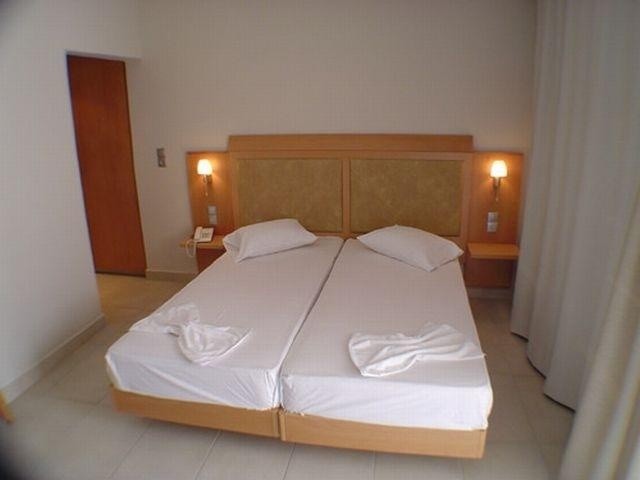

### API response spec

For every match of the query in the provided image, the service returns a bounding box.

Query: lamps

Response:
[197,159,212,184]
[490,160,507,189]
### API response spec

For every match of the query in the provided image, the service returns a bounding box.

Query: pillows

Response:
[356,225,465,272]
[222,217,320,264]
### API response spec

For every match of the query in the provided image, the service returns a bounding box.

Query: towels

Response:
[348,320,487,377]
[128,294,254,367]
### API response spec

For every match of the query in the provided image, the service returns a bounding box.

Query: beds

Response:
[104,236,345,438]
[278,237,494,459]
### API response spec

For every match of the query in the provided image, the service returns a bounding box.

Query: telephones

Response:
[193,226,214,242]
